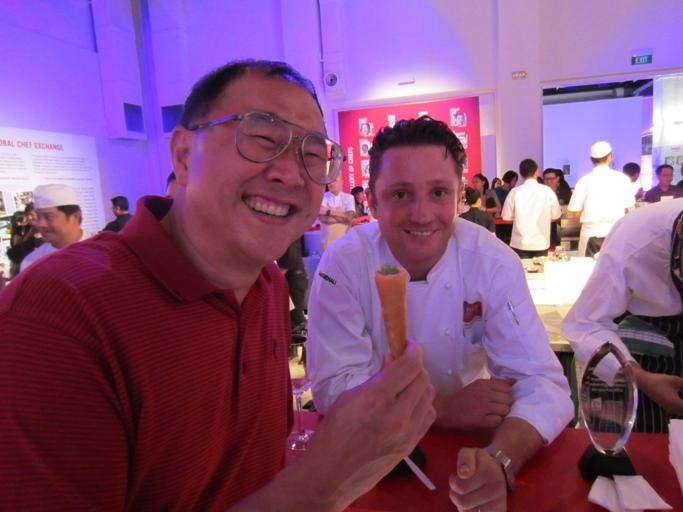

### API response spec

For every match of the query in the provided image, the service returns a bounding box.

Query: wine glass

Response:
[288,335,316,450]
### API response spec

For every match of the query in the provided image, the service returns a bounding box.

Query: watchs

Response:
[481,443,517,493]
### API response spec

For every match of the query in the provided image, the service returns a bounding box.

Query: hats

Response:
[33,183,80,209]
[590,141,611,159]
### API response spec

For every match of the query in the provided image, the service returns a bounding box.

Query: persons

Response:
[276,169,378,324]
[561,198,683,434]
[166,171,176,198]
[307,115,574,511]
[457,141,683,259]
[7,183,135,280]
[1,57,437,510]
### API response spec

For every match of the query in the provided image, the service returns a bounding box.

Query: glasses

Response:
[189,109,347,185]
